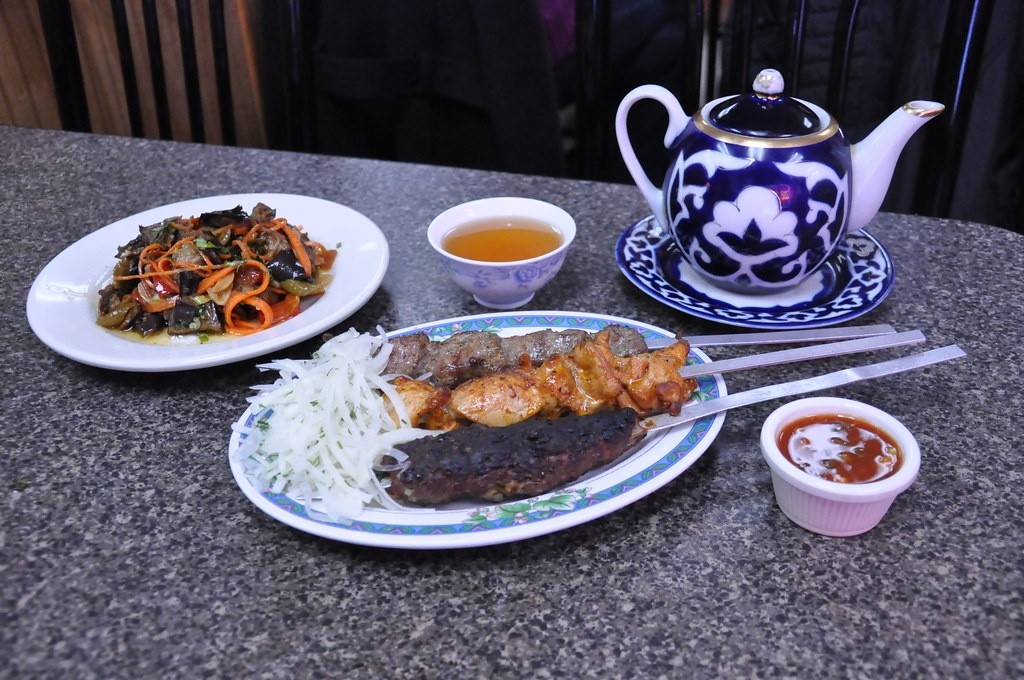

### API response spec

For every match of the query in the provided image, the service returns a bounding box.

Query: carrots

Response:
[114,218,311,334]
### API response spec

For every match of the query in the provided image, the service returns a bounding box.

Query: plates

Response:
[617,213,896,329]
[229,310,727,548]
[26,193,390,372]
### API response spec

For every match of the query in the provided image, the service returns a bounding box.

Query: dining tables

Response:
[0,126,1024,680]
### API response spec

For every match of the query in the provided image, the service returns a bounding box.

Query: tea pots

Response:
[616,68,945,295]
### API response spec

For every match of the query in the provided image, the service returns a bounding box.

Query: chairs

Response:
[693,0,989,217]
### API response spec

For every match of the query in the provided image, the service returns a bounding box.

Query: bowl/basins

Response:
[760,397,922,536]
[427,197,577,309]
